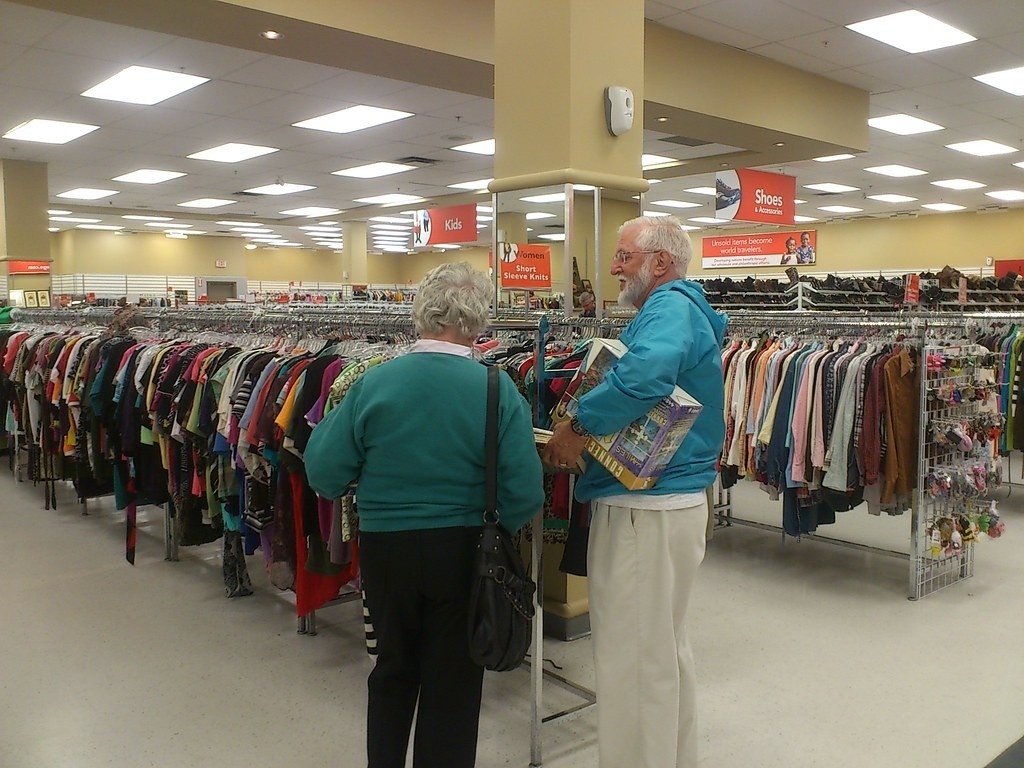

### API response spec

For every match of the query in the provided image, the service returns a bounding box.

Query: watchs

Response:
[570,415,593,438]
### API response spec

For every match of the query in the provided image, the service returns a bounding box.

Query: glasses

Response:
[614,250,658,264]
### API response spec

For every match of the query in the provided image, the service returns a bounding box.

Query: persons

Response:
[780,237,801,265]
[797,232,814,264]
[303,260,545,768]
[543,214,729,767]
[138,297,148,308]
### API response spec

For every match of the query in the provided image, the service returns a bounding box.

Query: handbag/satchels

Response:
[476,519,534,670]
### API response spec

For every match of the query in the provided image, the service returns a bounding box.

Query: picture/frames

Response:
[37,290,50,307]
[24,290,39,308]
[9,288,26,308]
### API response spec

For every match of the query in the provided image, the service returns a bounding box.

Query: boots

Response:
[784,267,800,291]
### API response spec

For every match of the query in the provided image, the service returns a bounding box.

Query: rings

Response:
[560,464,567,467]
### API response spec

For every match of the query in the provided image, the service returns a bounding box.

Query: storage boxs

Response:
[552,337,704,491]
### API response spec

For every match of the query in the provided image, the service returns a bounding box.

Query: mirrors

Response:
[564,184,599,319]
[599,189,644,319]
[490,184,566,320]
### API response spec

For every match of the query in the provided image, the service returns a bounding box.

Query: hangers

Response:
[6,294,1024,366]
